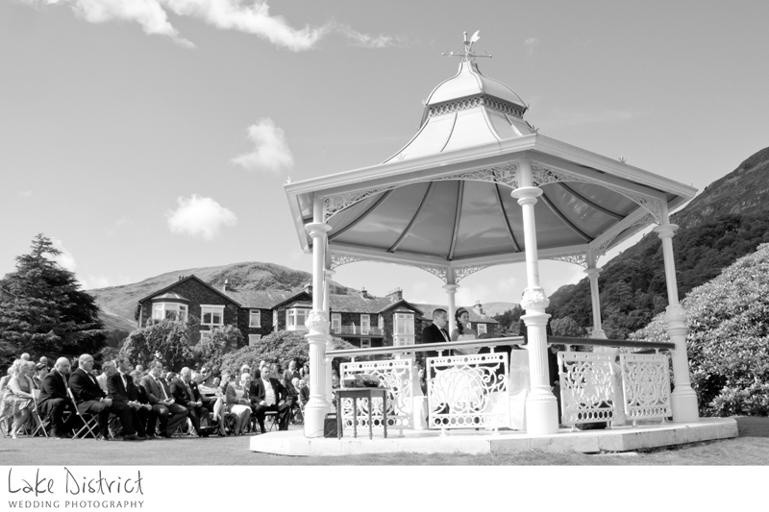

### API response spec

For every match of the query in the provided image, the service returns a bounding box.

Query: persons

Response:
[450,308,479,356]
[421,308,451,356]
[2,350,312,441]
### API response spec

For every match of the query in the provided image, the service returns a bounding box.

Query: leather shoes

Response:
[100,429,172,440]
[197,419,288,437]
[10,424,59,438]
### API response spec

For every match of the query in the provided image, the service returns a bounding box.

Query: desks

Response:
[334,387,389,439]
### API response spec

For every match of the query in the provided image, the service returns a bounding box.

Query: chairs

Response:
[16,388,278,439]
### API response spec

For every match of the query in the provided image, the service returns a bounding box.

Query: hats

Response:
[193,367,212,382]
[240,364,252,374]
